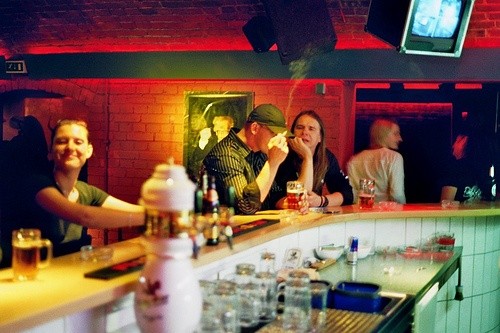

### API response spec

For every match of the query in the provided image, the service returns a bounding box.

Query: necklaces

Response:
[55,181,67,196]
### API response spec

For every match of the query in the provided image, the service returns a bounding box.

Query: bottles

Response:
[347,237,359,266]
[189,165,219,246]
[486,159,497,201]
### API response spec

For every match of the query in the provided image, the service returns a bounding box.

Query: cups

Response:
[194,247,328,333]
[358,177,376,210]
[11,229,52,283]
[286,181,305,214]
[439,186,457,205]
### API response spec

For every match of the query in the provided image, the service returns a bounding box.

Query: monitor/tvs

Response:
[365,0,475,58]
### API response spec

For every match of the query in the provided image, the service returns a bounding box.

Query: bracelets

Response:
[319,195,323,208]
[322,194,329,207]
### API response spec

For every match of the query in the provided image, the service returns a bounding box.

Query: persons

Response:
[442,134,478,201]
[31,118,147,262]
[347,118,407,204]
[201,102,295,216]
[269,110,354,215]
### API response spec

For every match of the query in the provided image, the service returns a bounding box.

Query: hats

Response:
[248,104,296,136]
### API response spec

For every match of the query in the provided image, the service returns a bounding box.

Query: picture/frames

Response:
[182,90,255,183]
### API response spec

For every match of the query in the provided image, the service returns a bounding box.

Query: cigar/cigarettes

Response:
[281,130,287,137]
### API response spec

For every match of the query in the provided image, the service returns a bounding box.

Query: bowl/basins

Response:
[316,246,342,261]
[357,247,372,260]
